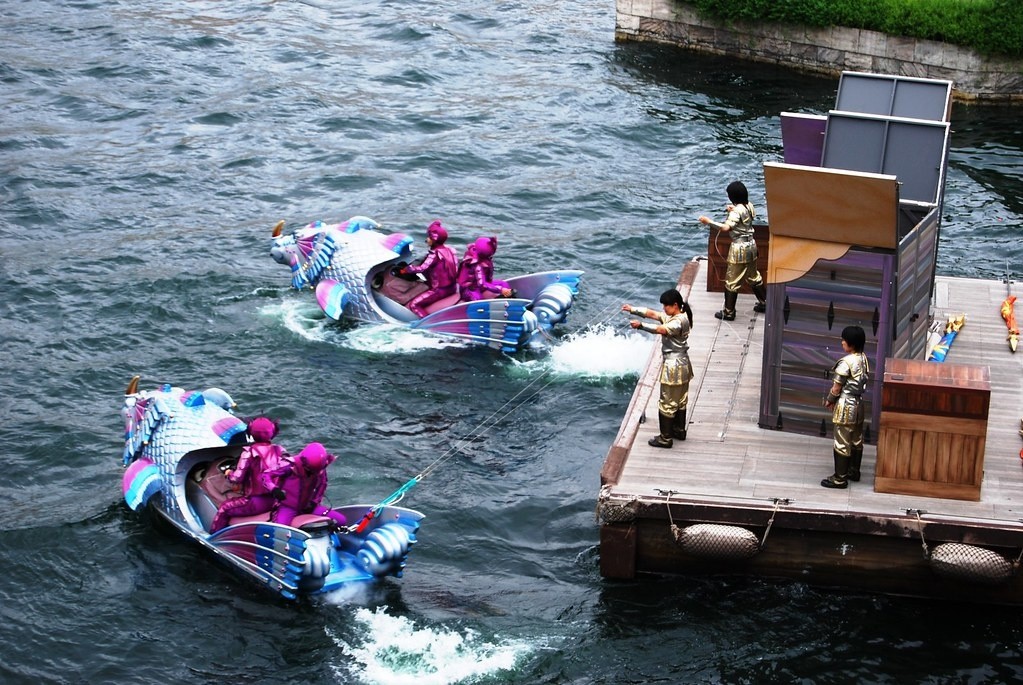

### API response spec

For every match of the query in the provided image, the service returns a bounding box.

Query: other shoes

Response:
[848,477,860,481]
[821,479,846,488]
[649,439,672,448]
[716,312,734,321]
[673,436,685,440]
[753,305,765,312]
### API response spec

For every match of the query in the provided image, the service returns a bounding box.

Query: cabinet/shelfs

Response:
[707,220,769,295]
[875,357,991,500]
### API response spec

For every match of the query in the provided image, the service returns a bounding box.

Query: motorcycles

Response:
[269,214,586,359]
[120,375,427,614]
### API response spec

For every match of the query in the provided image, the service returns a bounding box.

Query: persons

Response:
[820,325,870,489]
[621,289,694,449]
[209,417,292,535]
[400,220,459,319]
[698,181,766,321]
[455,235,518,301]
[261,442,348,526]
